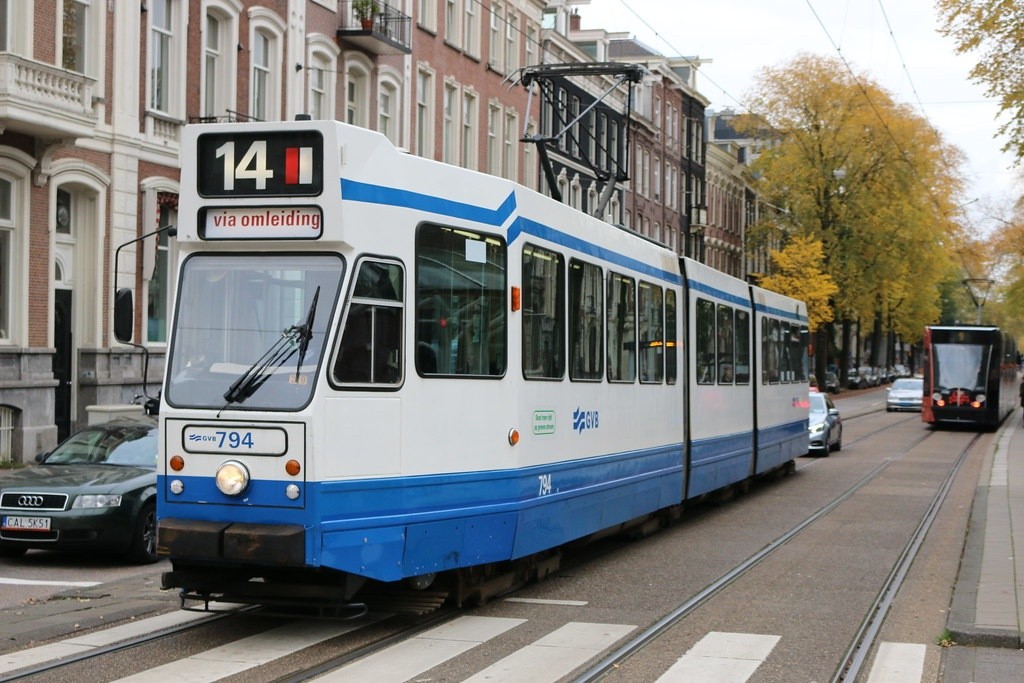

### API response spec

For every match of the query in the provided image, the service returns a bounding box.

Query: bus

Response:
[113,56,816,622]
[908,275,1024,434]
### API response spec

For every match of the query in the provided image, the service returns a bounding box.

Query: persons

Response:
[344,261,400,382]
[809,369,818,386]
[721,366,733,381]
[762,370,776,382]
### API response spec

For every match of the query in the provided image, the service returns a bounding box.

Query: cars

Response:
[0,409,162,566]
[805,390,843,459]
[884,377,924,413]
[809,363,913,396]
